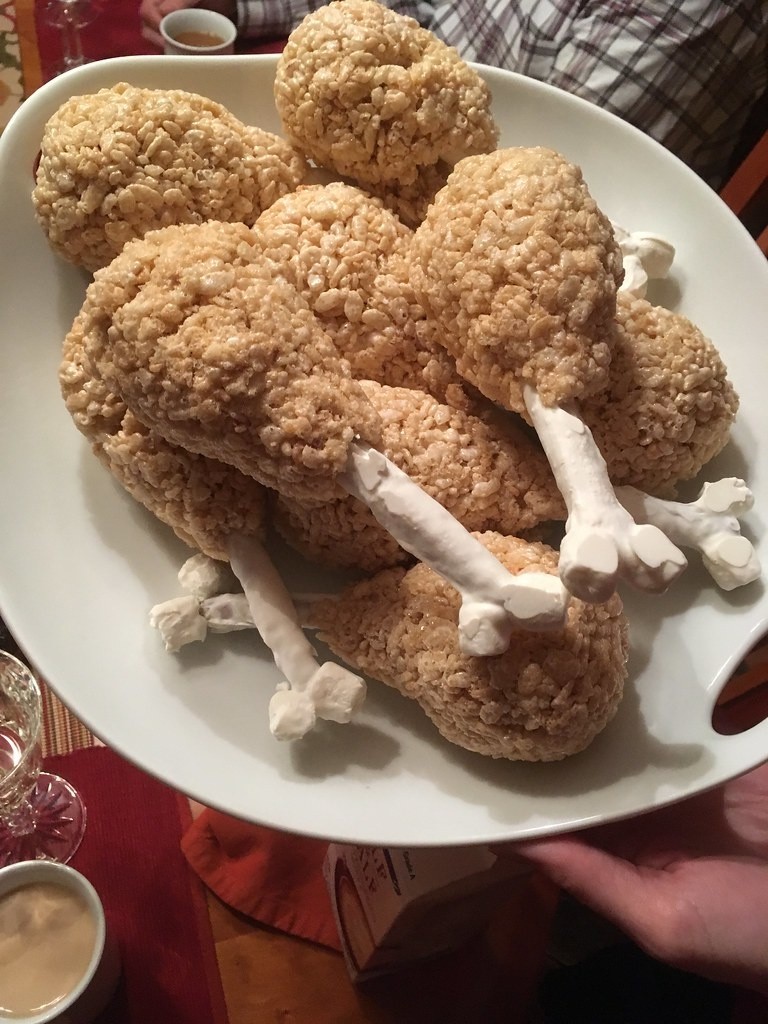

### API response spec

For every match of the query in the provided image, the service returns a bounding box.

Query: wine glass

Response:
[1,649,86,870]
[38,0,103,78]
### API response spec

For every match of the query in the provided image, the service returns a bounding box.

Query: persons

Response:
[136,2,767,219]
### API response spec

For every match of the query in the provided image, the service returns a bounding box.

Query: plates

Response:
[0,55,765,849]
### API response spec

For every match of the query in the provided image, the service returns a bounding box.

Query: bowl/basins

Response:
[0,859,122,1024]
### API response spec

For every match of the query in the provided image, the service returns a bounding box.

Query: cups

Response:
[159,8,237,57]
